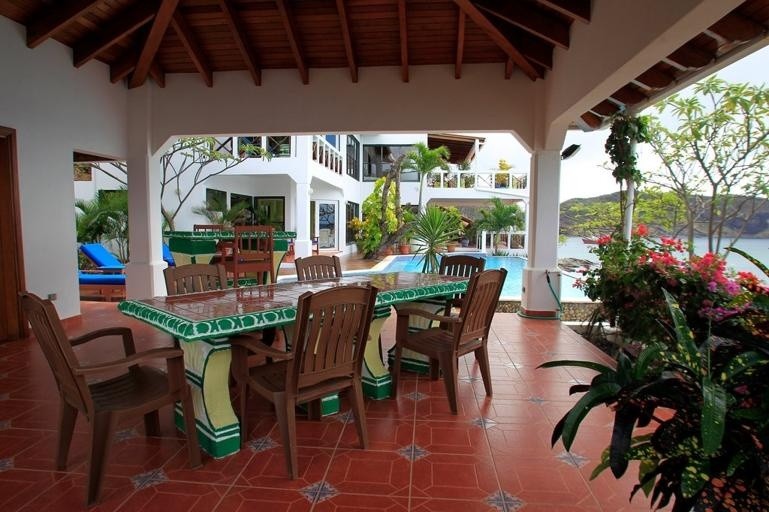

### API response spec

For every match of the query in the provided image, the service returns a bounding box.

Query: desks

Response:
[118,270,471,345]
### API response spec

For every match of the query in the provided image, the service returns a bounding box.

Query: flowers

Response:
[571,222,769,425]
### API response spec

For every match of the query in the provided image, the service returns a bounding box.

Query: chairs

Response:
[162,264,277,347]
[16,289,199,508]
[390,267,509,420]
[77,242,127,301]
[209,224,276,285]
[294,254,343,280]
[227,283,380,479]
[438,254,487,280]
